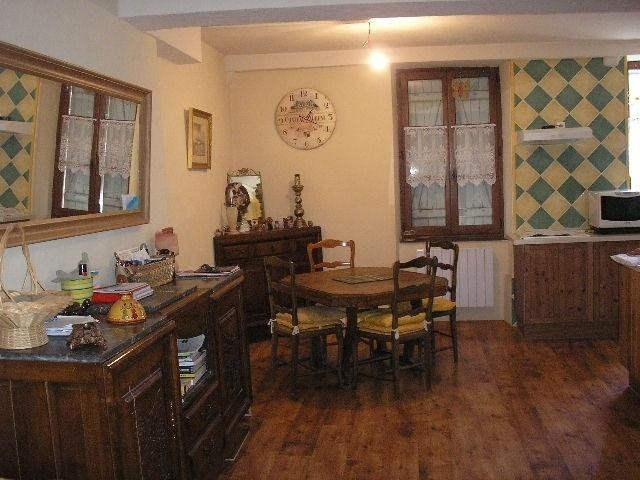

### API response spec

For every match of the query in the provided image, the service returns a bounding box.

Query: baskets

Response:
[0,220,74,350]
[114,246,176,291]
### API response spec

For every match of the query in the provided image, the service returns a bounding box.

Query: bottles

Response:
[227,204,239,234]
[274,220,280,230]
[108,293,147,323]
[237,215,251,233]
[79,262,87,277]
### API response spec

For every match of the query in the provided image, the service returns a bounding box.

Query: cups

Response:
[61,278,94,306]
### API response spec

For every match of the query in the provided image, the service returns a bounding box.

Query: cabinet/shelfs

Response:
[214,226,322,343]
[0,267,253,480]
[513,234,640,341]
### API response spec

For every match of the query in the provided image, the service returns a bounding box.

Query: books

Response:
[176,265,241,277]
[93,282,154,304]
[177,333,207,386]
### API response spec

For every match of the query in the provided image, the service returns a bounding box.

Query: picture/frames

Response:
[187,107,213,170]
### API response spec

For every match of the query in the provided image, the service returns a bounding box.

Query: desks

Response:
[608,252,640,395]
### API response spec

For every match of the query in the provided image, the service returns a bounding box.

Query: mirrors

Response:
[227,169,265,225]
[0,40,152,248]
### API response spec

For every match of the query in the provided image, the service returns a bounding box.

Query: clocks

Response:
[274,88,336,150]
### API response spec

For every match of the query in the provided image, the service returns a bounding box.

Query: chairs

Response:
[262,237,470,396]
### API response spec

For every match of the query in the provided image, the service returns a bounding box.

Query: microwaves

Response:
[588,190,640,233]
